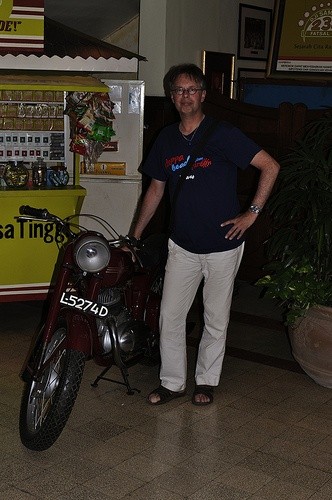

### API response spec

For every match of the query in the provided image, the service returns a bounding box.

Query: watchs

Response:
[249,205,263,214]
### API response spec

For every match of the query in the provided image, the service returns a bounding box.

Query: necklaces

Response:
[180,114,205,148]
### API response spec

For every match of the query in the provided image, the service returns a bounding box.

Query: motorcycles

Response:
[17,205,201,452]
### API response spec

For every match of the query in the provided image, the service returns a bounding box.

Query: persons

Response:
[115,64,281,406]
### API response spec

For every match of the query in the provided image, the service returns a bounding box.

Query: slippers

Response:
[191,385,215,405]
[146,384,186,405]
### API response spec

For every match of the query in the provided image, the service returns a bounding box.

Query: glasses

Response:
[170,87,202,95]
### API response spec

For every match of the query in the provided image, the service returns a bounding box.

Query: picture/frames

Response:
[238,77,332,110]
[200,49,236,101]
[236,2,274,72]
[265,0,332,78]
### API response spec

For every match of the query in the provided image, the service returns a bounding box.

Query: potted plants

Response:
[254,109,332,389]
